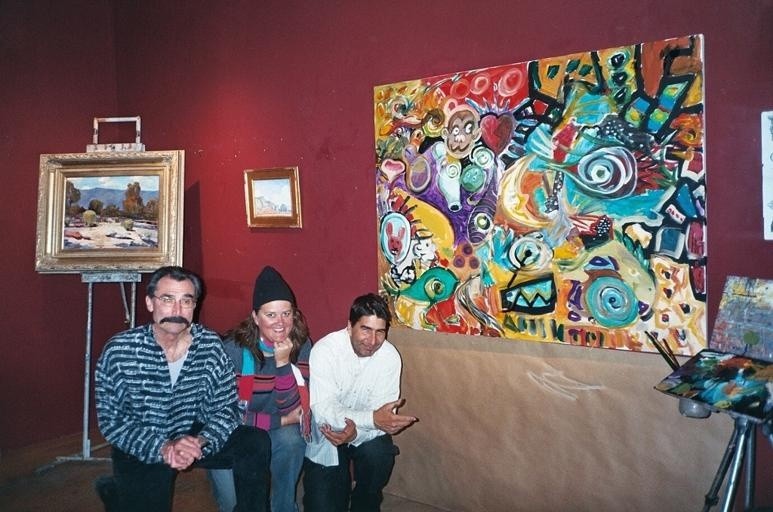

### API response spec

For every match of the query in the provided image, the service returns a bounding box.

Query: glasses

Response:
[151,294,195,308]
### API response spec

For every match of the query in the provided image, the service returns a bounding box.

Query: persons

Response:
[305,295,419,511]
[206,265,309,512]
[92,266,273,511]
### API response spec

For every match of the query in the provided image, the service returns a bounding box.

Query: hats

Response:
[250,264,297,313]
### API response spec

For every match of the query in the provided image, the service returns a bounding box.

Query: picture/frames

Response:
[32,150,186,278]
[244,165,304,233]
